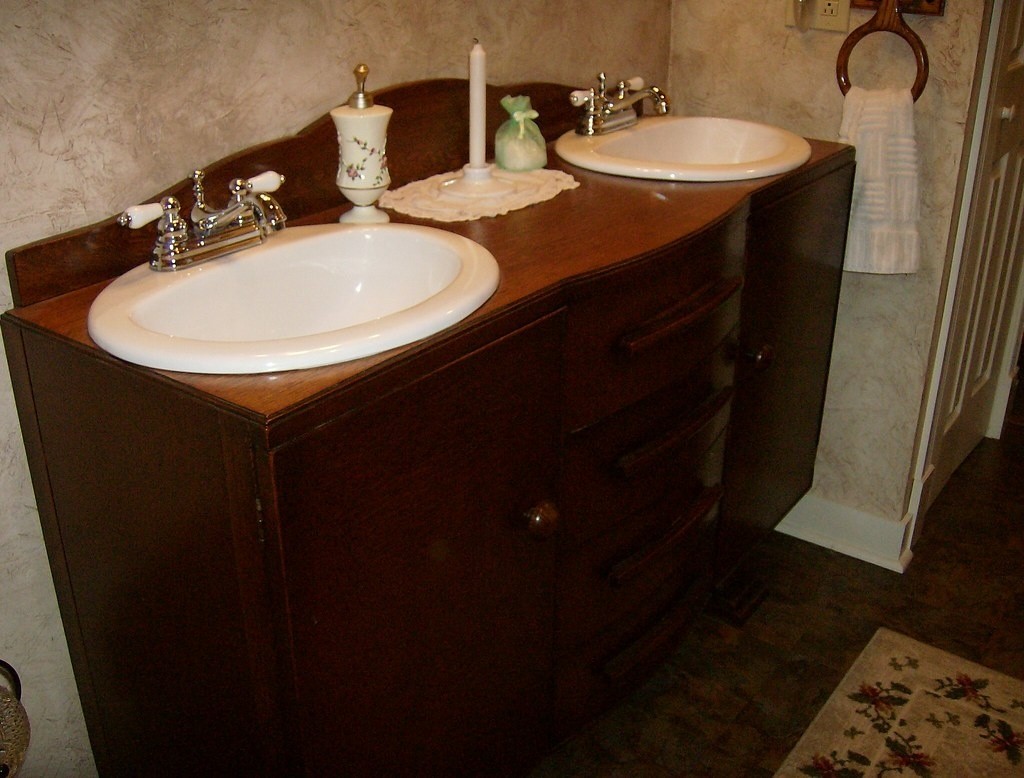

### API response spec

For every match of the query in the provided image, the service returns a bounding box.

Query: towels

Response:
[836,84,922,275]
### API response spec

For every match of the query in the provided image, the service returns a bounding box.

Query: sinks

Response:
[86,222,501,375]
[553,114,812,183]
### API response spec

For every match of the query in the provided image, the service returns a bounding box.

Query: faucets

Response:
[569,71,671,137]
[116,167,286,273]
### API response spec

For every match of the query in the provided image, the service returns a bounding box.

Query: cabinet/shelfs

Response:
[254,161,857,776]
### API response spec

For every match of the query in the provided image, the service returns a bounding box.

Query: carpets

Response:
[773,623,1024,778]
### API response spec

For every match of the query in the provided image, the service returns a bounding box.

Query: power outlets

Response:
[808,0,851,33]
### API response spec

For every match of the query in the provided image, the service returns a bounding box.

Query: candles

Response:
[468,36,486,166]
